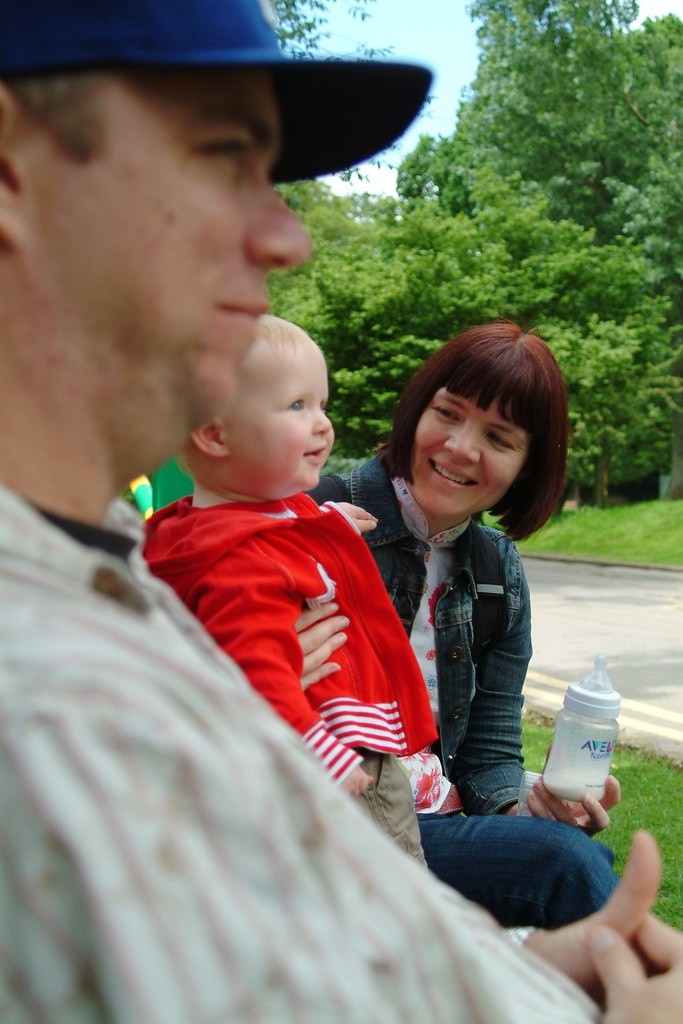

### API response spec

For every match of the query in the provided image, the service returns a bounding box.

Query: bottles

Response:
[542,655,622,803]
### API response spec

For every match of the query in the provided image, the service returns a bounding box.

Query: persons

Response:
[320,314,621,930]
[143,314,438,871]
[0,1,683,1024]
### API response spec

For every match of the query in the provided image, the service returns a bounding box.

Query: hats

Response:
[0,0,432,182]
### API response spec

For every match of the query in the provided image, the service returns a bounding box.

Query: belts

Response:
[434,784,464,815]
